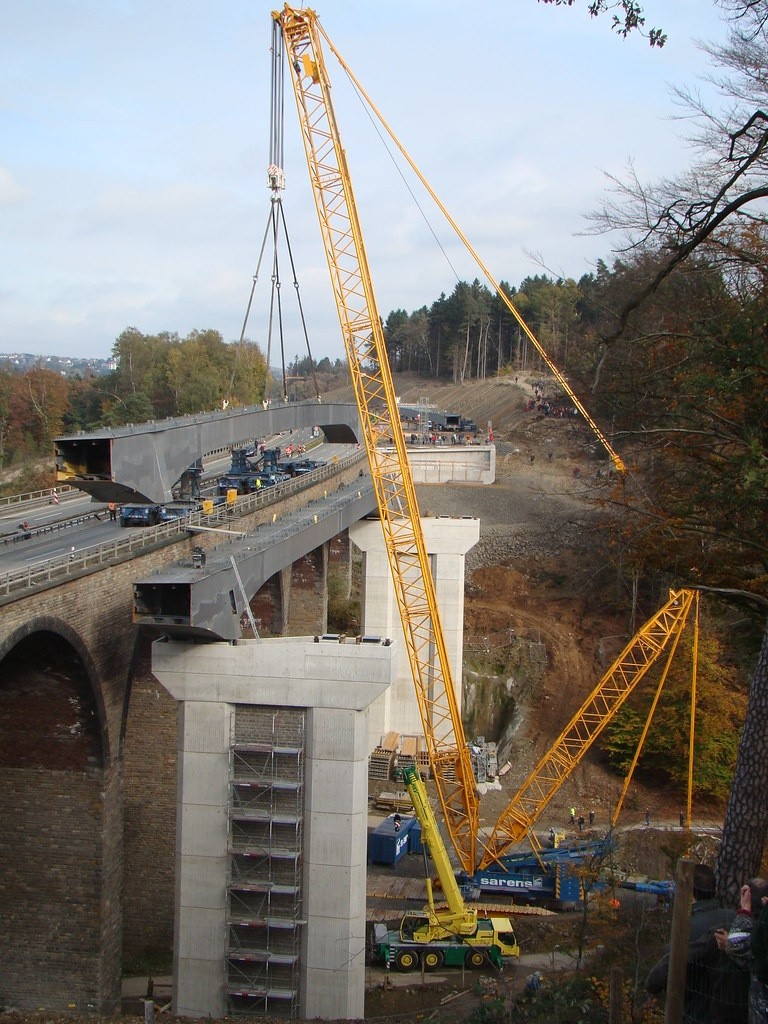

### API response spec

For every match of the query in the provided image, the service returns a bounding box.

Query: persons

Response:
[589,811,595,825]
[680,812,685,827]
[256,477,261,491]
[527,380,578,418]
[568,807,575,825]
[548,451,552,461]
[645,864,768,1024]
[410,433,494,446]
[645,807,650,825]
[286,444,307,459]
[24,522,29,530]
[528,971,541,1003]
[53,491,58,503]
[578,814,584,830]
[258,440,266,456]
[516,376,518,383]
[530,453,535,465]
[357,444,360,449]
[108,501,117,521]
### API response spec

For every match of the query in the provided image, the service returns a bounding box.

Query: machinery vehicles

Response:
[373,763,520,971]
[266,1,700,918]
